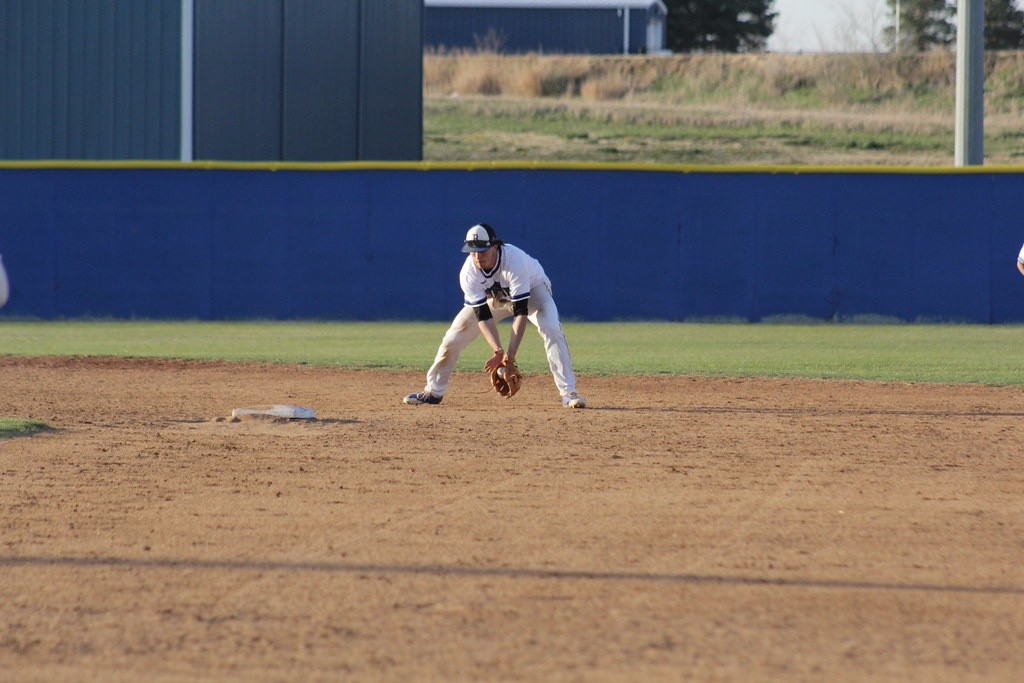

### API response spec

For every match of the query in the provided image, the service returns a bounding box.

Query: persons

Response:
[403,224,585,407]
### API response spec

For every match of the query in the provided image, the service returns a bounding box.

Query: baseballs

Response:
[497,367,506,375]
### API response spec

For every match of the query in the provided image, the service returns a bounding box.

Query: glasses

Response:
[466,241,488,247]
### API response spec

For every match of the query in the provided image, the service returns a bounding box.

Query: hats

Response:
[461,223,496,252]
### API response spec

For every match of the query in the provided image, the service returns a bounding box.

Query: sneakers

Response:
[562,392,586,408]
[404,392,440,404]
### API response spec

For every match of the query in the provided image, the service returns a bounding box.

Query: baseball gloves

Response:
[477,359,525,401]
